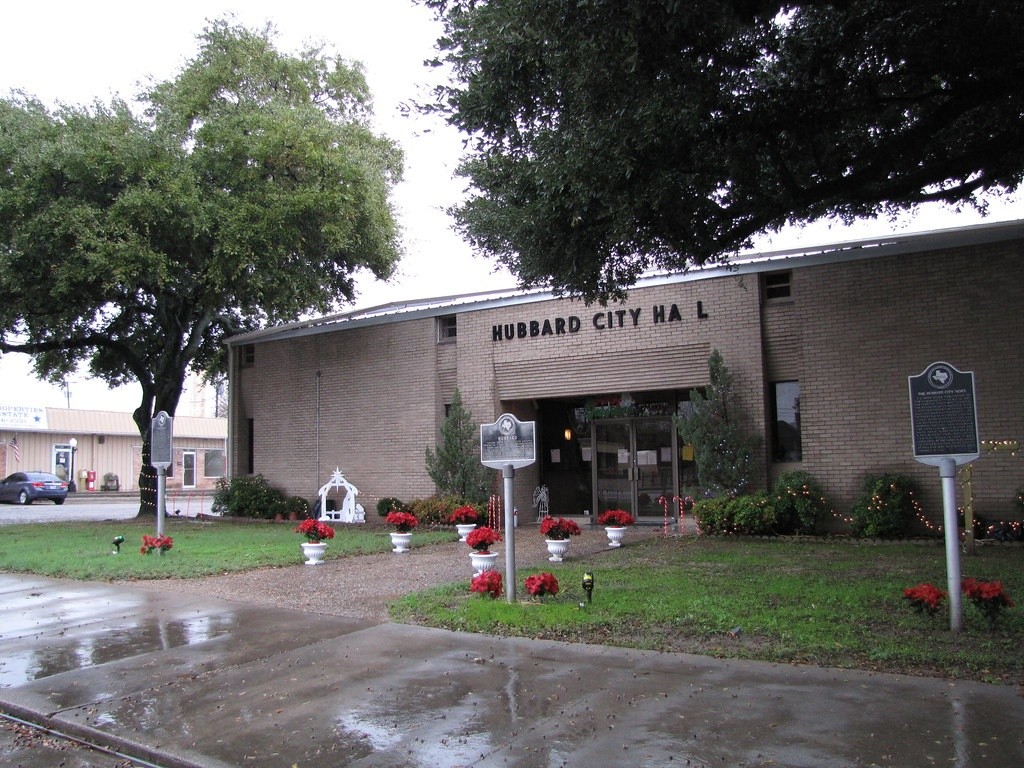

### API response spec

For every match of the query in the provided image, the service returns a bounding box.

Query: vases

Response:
[301,542,327,558]
[470,551,499,572]
[455,524,477,536]
[390,532,412,547]
[605,526,626,540]
[545,538,570,554]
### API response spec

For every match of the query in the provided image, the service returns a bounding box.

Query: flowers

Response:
[598,509,635,529]
[299,519,334,543]
[450,505,478,524]
[386,511,420,534]
[466,526,502,554]
[540,514,581,540]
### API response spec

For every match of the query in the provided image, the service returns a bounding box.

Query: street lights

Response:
[68,437,77,492]
[52,382,78,408]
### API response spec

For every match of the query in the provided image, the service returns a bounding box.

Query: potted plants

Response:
[269,495,306,523]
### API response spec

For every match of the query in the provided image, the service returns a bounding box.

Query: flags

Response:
[9,436,21,463]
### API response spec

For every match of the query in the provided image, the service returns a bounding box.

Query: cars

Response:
[0,471,70,504]
